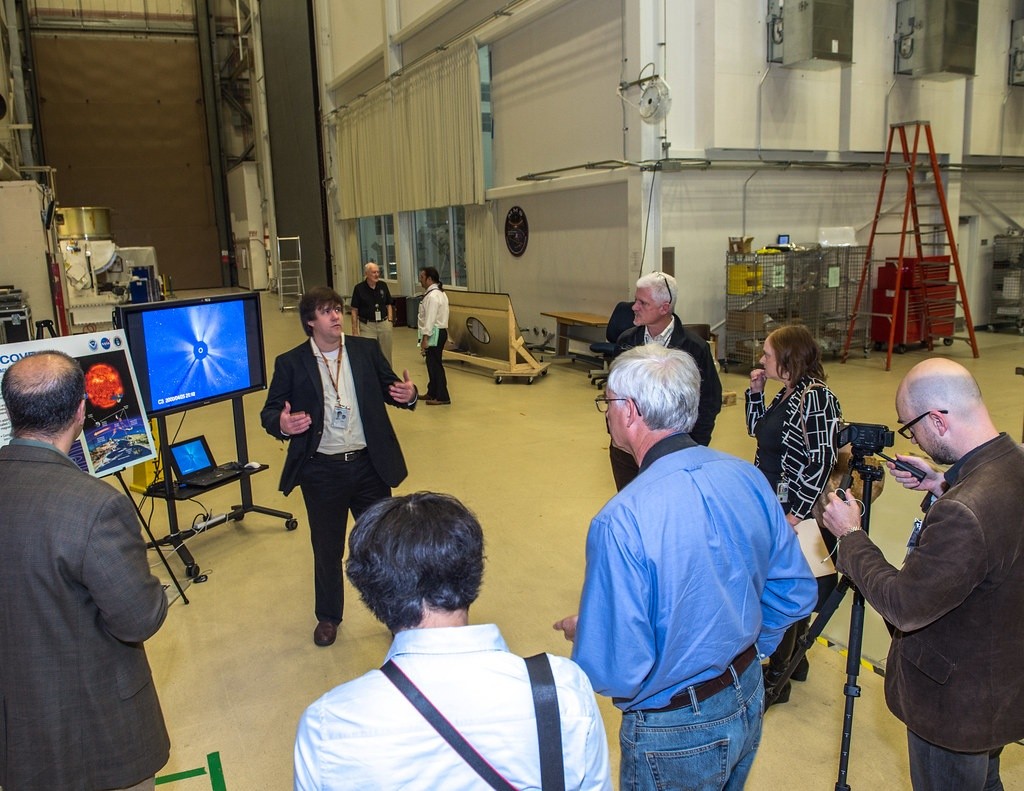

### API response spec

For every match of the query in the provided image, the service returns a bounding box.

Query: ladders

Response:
[843,121,979,371]
[276,236,306,313]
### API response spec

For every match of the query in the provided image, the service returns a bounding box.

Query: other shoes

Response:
[426,399,450,405]
[418,394,430,400]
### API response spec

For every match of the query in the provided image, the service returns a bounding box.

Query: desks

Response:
[541,311,611,370]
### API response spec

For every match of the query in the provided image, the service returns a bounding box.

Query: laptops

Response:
[169,434,243,489]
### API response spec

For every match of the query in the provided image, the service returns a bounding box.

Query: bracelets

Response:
[423,335,427,336]
[388,319,393,322]
[838,526,861,542]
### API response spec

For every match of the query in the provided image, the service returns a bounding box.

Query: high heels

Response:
[763,670,791,703]
[762,655,809,681]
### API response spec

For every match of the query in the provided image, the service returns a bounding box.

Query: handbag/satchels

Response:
[811,442,886,528]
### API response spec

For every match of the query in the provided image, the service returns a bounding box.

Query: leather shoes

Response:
[314,621,337,645]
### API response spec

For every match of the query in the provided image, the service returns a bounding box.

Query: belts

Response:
[631,644,757,712]
[369,317,388,322]
[315,447,368,462]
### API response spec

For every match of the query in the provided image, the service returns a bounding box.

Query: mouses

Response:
[244,462,261,469]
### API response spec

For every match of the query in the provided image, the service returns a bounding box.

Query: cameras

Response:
[836,422,894,449]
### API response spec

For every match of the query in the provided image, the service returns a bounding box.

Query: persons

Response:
[745,324,843,704]
[336,411,342,419]
[349,262,394,372]
[606,269,722,493]
[260,286,419,647]
[822,356,1024,791]
[292,490,614,791]
[416,265,451,405]
[552,342,818,791]
[779,485,787,493]
[0,350,171,791]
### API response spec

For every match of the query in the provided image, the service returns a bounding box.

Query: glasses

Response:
[898,411,948,439]
[652,271,672,304]
[595,392,642,416]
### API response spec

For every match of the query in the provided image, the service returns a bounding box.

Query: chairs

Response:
[589,302,635,390]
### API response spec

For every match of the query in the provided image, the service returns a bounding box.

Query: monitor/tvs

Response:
[115,291,267,420]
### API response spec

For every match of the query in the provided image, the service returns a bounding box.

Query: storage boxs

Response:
[728,254,784,296]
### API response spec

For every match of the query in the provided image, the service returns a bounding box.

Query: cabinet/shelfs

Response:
[988,235,1024,333]
[724,245,873,371]
[872,256,957,352]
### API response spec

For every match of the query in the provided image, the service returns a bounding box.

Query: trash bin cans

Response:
[391,295,406,326]
[408,296,422,328]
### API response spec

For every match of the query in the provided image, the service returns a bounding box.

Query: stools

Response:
[35,319,56,340]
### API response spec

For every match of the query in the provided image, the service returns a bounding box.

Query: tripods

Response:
[762,447,927,791]
